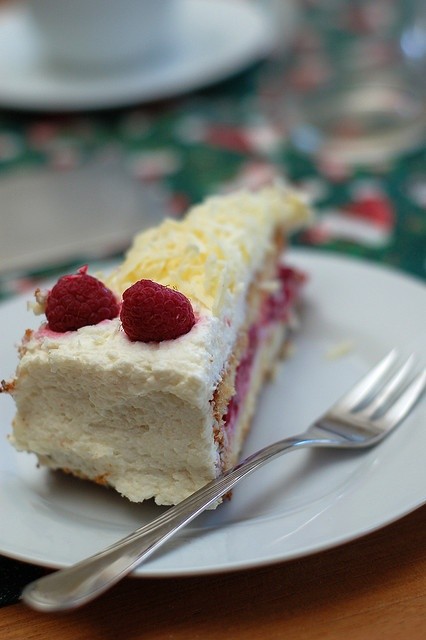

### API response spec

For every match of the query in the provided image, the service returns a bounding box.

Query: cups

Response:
[268,2,426,160]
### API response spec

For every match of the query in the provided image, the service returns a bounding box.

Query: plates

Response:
[2,2,277,112]
[1,248,424,579]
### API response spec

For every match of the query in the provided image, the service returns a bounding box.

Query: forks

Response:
[18,349,424,614]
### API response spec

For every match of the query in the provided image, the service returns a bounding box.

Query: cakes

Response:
[0,185,310,512]
